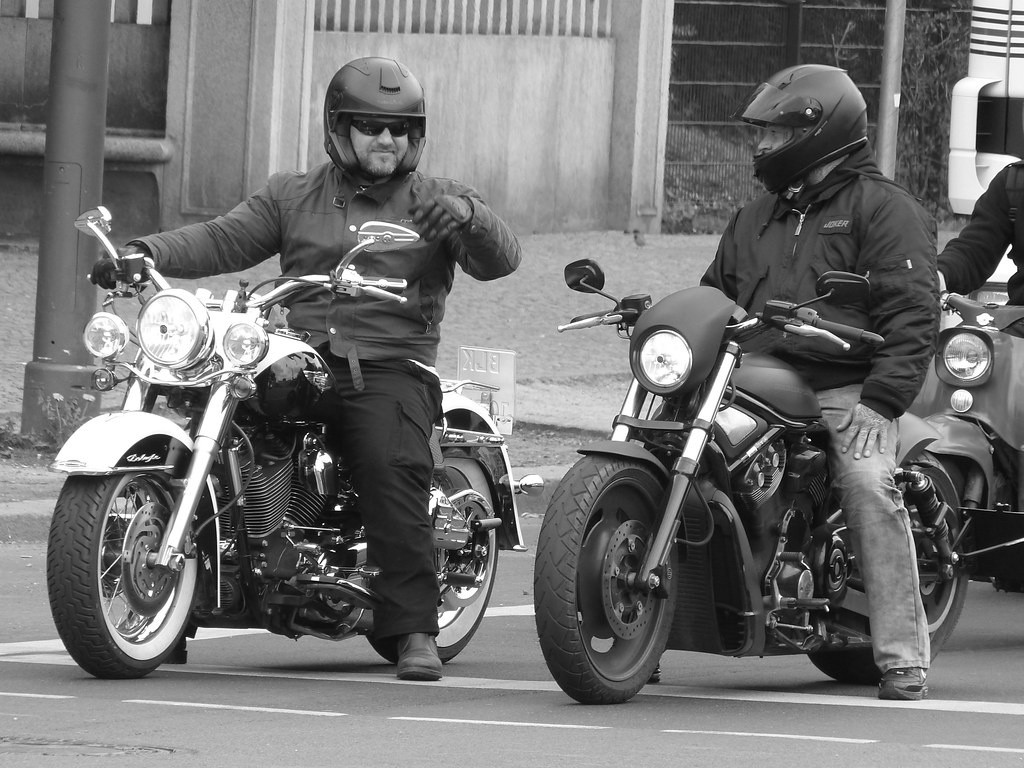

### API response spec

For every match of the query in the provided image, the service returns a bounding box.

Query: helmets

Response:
[323,55,428,184]
[728,64,868,194]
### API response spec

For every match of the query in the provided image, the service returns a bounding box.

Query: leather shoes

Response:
[396,632,445,681]
[163,636,188,665]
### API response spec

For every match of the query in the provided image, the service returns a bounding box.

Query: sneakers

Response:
[878,667,929,700]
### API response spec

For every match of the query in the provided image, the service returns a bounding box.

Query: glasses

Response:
[350,118,414,137]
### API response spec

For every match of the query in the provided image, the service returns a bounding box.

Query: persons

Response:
[935,159,1024,336]
[90,56,523,680]
[698,65,941,701]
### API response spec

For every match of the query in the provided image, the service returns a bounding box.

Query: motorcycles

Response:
[535,258,969,704]
[907,289,1024,592]
[47,206,527,680]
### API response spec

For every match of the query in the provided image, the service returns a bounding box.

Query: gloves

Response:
[409,195,475,243]
[91,240,152,290]
